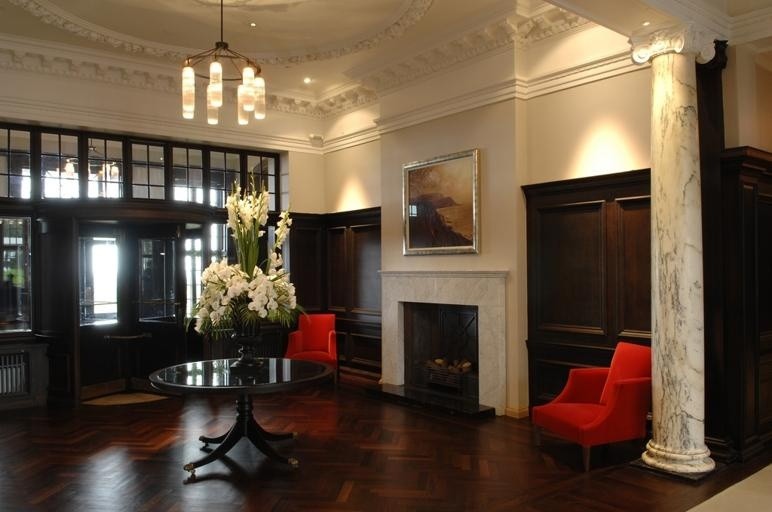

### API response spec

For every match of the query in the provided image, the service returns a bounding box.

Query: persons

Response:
[4,273,23,318]
[141,259,152,295]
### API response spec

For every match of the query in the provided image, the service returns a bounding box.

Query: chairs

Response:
[532,342,652,472]
[284,314,339,385]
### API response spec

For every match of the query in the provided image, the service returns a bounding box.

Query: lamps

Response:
[65,138,119,180]
[181,0,266,125]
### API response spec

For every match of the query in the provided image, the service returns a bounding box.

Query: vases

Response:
[230,329,265,371]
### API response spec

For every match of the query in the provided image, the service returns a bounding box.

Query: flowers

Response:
[184,167,310,342]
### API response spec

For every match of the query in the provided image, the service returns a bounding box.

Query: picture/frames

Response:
[402,148,481,256]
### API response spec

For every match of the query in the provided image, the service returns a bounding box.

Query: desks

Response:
[149,358,334,475]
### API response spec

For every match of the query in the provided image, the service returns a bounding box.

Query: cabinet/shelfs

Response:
[521,147,771,464]
[289,208,381,392]
